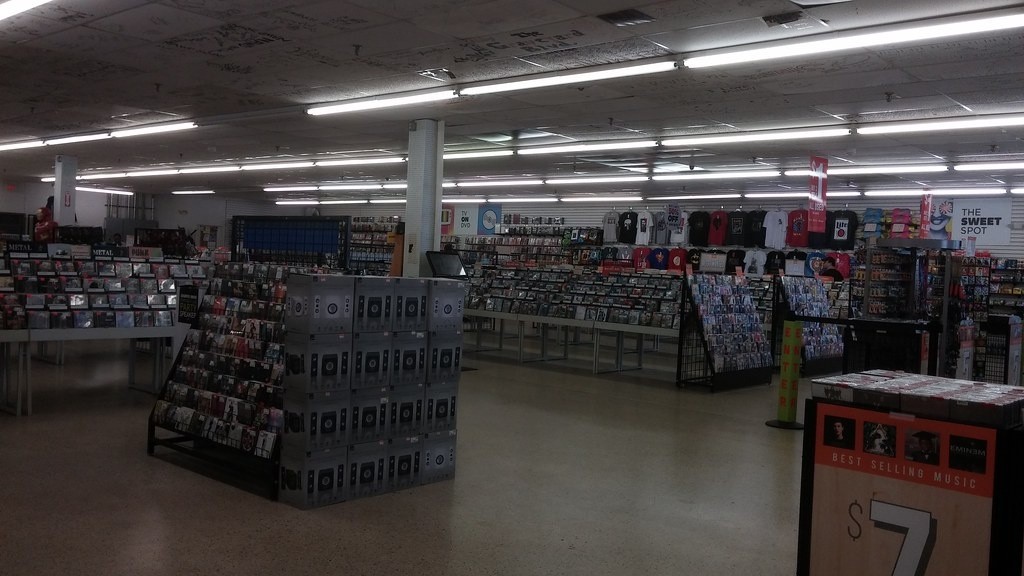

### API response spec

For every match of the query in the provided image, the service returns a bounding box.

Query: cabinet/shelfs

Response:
[441,215,1024,576]
[0,214,405,502]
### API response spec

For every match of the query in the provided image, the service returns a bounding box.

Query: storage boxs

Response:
[278,271,465,509]
[796,396,1024,576]
